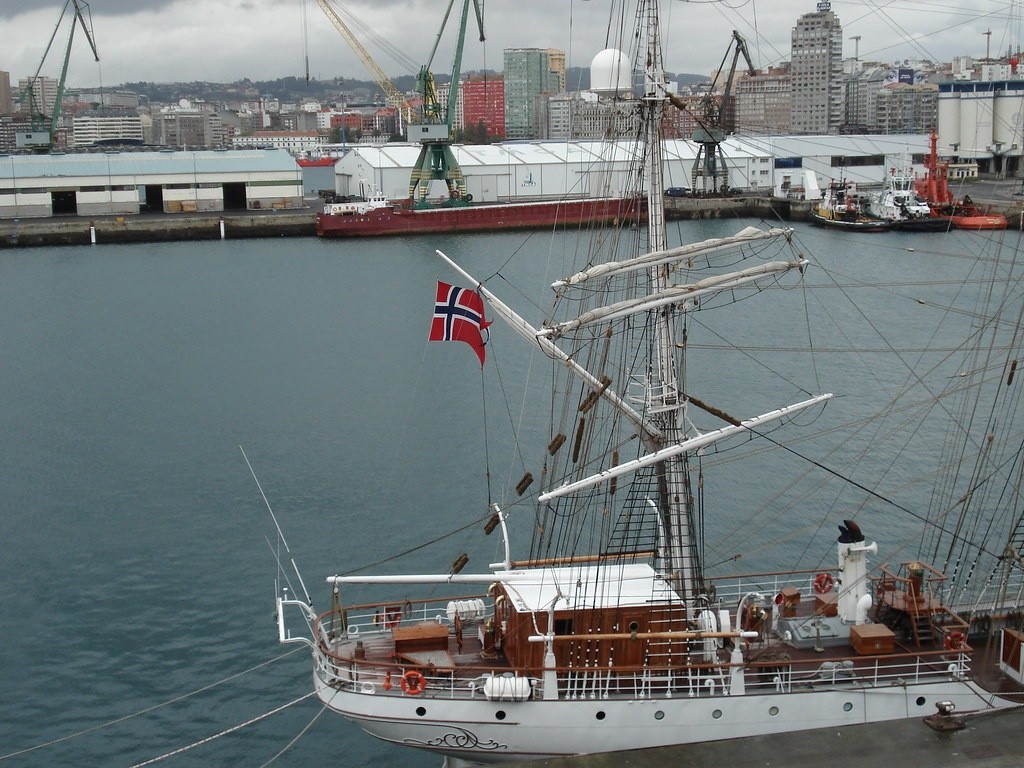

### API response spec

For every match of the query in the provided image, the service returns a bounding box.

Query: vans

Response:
[663,186,685,197]
[685,187,697,196]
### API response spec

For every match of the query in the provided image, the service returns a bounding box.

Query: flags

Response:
[426,280,495,369]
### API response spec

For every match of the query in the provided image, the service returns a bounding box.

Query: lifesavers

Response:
[400,671,425,694]
[945,632,966,650]
[814,574,834,594]
[381,610,402,628]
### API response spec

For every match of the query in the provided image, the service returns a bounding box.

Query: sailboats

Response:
[244,0,1022,759]
